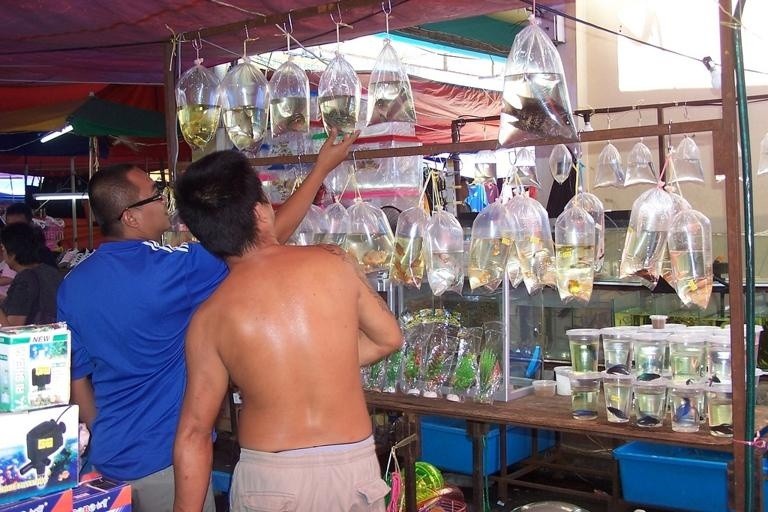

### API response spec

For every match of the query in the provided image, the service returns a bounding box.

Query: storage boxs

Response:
[0,318,133,512]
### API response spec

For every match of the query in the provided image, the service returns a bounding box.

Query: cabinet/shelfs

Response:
[160,0,767,512]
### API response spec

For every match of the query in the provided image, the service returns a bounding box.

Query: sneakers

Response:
[56,247,95,269]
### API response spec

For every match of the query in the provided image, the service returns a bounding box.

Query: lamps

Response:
[39,126,75,142]
[32,189,90,203]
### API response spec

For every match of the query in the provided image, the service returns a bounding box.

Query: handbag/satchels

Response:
[27,304,46,324]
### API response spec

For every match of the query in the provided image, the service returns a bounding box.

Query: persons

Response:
[172,150,404,510]
[0,220,61,325]
[56,124,362,511]
[1,202,61,299]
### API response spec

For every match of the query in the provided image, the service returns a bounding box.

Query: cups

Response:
[567,323,765,441]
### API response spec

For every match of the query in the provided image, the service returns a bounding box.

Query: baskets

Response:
[421,496,468,512]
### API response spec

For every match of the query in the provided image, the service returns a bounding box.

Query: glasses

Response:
[117,180,165,220]
